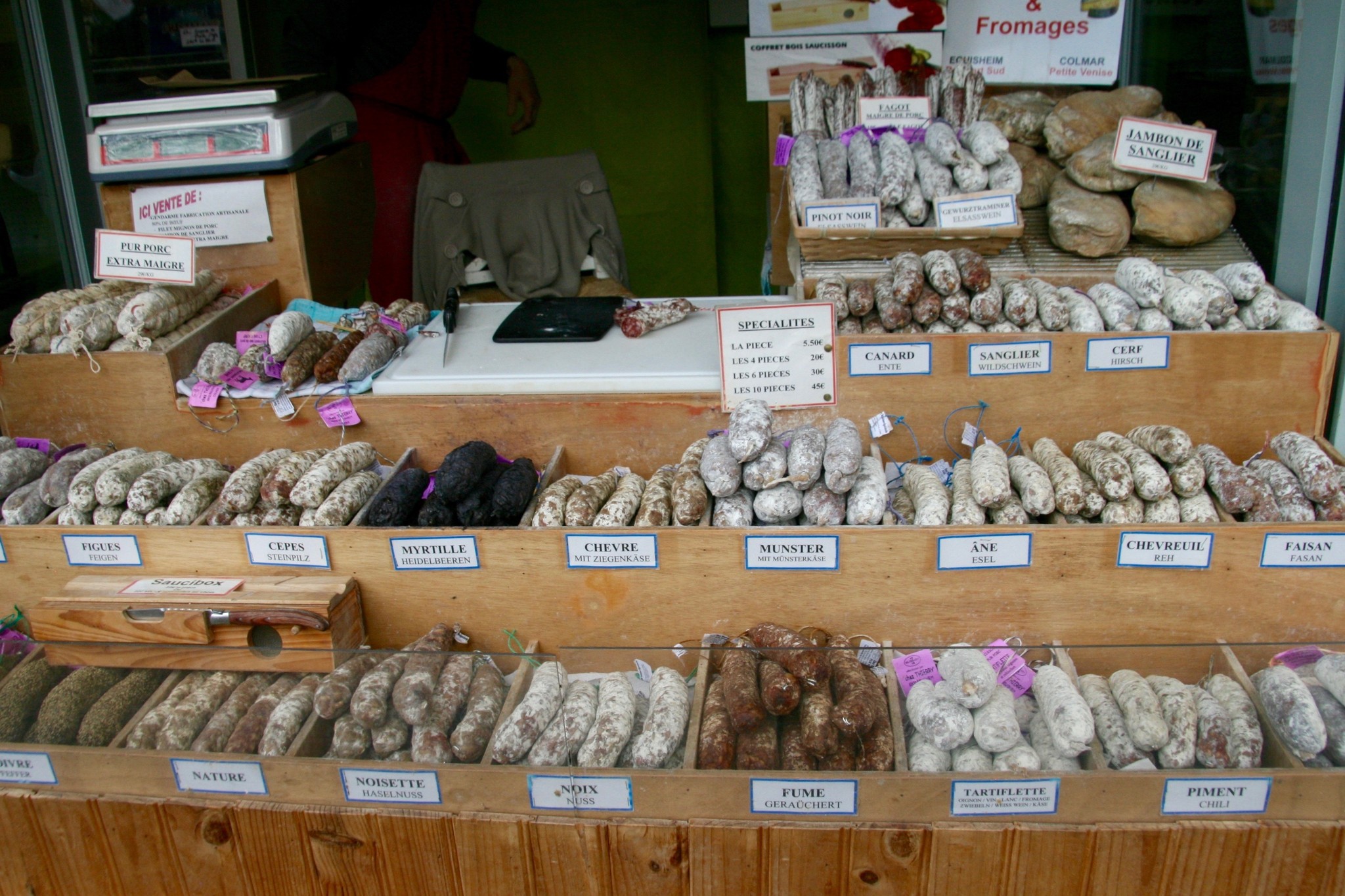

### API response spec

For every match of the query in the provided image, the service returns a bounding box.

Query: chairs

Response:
[455,244,635,305]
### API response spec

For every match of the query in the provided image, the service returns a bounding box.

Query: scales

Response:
[84,70,359,178]
